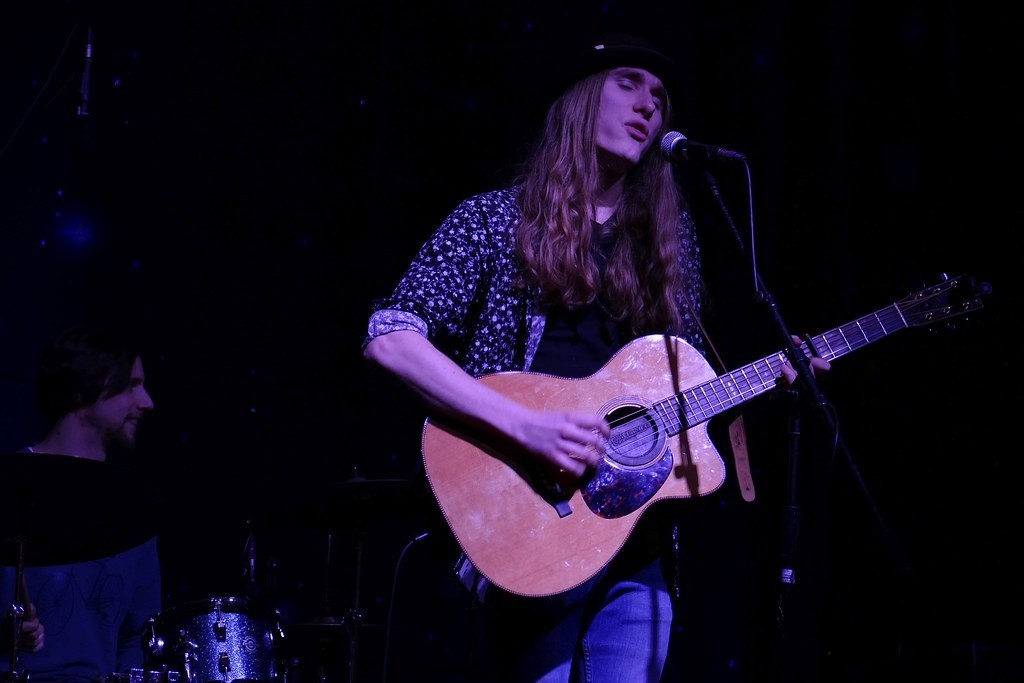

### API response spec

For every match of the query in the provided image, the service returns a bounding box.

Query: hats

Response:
[565,32,685,89]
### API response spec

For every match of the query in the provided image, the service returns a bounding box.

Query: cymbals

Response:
[283,614,377,627]
[330,477,412,488]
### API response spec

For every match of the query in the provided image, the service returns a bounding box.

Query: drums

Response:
[138,595,282,683]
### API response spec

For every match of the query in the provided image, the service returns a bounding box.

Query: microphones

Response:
[248,538,255,582]
[660,131,749,164]
[77,25,93,116]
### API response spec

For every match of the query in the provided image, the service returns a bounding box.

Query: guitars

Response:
[417,264,994,614]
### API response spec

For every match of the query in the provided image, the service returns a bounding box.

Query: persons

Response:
[362,33,831,683]
[0,327,168,683]
